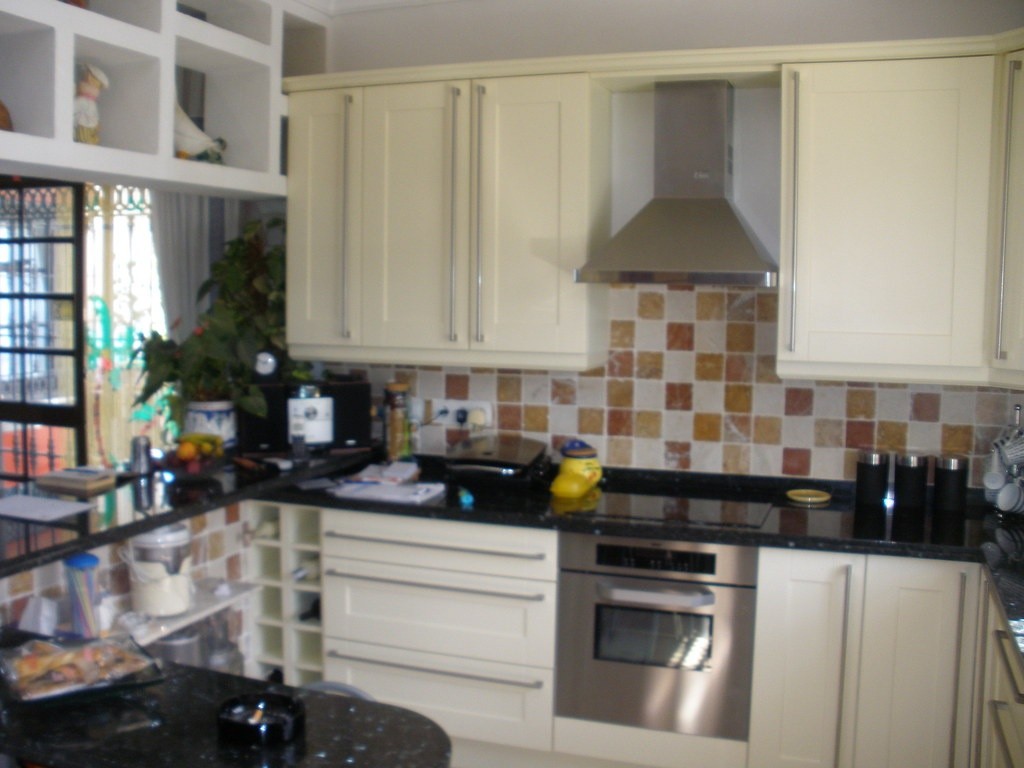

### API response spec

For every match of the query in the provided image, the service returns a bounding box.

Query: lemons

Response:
[550,472,589,498]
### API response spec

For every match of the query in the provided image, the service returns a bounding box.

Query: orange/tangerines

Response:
[176,442,196,460]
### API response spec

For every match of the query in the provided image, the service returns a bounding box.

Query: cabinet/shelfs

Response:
[362,54,611,372]
[248,498,557,754]
[746,545,867,768]
[979,562,1024,768]
[284,70,363,363]
[774,35,1000,388]
[0,0,334,201]
[0,496,265,682]
[854,555,982,768]
[993,26,1024,391]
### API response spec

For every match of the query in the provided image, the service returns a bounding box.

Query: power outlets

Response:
[432,397,493,428]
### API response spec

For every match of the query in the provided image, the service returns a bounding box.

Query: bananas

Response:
[174,433,224,458]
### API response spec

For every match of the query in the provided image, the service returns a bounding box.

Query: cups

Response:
[981,471,1005,501]
[997,476,1024,513]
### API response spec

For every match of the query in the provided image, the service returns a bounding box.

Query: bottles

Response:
[382,382,414,462]
[291,555,320,583]
[62,551,101,637]
[298,597,320,623]
[253,518,279,538]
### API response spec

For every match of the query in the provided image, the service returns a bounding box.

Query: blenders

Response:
[125,523,194,618]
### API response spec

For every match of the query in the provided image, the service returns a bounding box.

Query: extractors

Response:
[566,190,778,290]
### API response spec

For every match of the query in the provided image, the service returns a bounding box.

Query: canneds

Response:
[382,384,412,460]
[560,448,603,490]
[131,435,150,474]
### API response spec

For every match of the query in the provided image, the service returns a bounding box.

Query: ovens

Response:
[551,491,773,745]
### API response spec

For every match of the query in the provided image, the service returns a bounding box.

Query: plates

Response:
[786,488,833,504]
